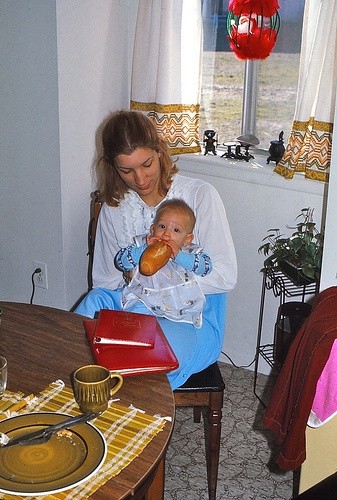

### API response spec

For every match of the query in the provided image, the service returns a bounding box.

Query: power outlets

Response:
[31,261,48,288]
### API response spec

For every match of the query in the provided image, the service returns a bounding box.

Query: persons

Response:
[114,198,212,329]
[68,110,238,391]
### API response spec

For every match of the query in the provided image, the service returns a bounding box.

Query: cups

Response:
[73,365,123,414]
[0,356,8,398]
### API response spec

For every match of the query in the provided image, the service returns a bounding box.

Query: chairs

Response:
[88,198,226,500]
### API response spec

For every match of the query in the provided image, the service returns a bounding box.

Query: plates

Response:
[0,412,108,496]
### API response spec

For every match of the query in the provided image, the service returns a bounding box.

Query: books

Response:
[85,309,179,378]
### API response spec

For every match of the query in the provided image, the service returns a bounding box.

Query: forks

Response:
[18,413,100,446]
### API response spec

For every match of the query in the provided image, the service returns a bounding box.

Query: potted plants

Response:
[257,208,321,286]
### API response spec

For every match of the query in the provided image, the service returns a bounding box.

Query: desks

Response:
[0,301,176,500]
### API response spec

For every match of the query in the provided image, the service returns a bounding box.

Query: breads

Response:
[139,240,172,276]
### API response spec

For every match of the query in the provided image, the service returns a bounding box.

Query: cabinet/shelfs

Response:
[253,268,316,410]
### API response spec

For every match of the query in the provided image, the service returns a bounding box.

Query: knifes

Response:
[0,413,99,448]
